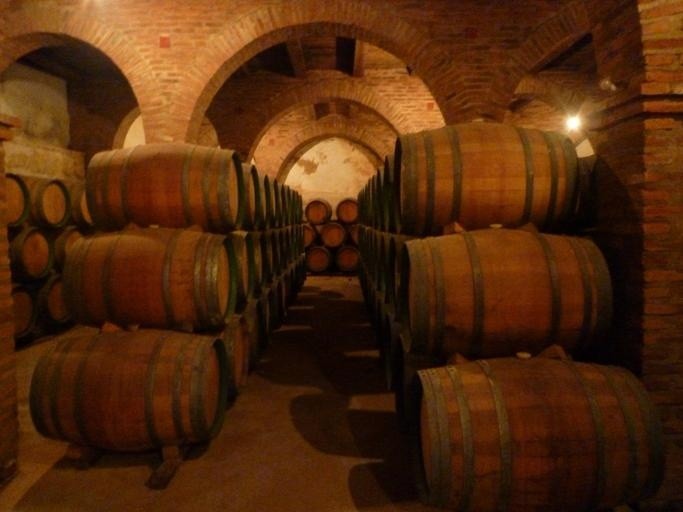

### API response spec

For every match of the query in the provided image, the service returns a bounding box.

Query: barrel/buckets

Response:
[0,125,666,512]
